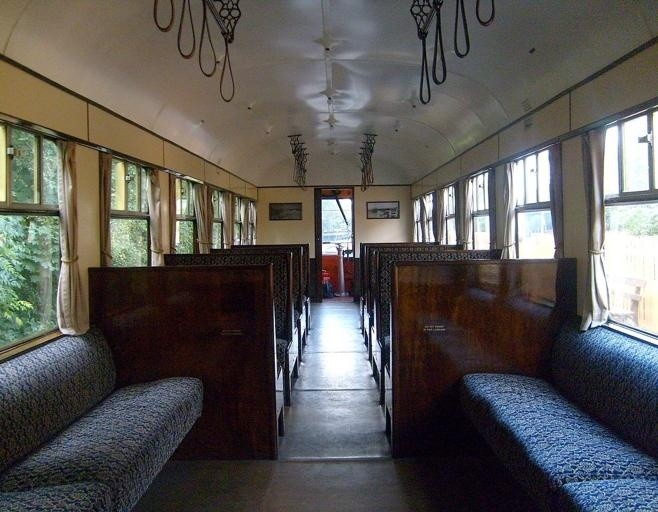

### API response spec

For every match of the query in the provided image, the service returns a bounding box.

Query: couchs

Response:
[1,324,204,511]
[460,319,658,512]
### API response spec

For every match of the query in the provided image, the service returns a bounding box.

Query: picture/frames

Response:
[269,202,302,220]
[367,201,400,219]
